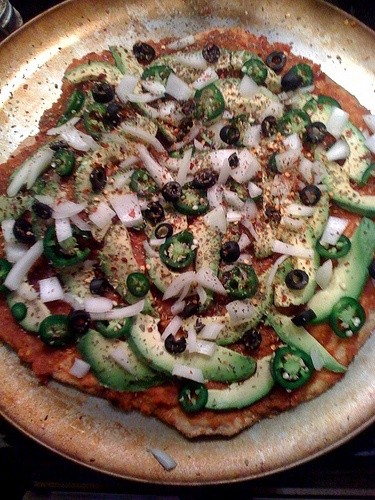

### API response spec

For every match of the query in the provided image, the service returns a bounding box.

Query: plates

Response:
[0,0,375,488]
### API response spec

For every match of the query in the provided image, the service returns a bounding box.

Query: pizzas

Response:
[0,27,375,439]
